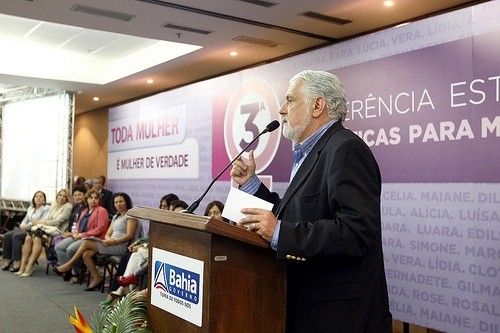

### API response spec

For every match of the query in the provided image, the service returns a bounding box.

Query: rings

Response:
[251,222,256,230]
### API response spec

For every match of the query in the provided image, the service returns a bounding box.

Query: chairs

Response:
[86,253,121,296]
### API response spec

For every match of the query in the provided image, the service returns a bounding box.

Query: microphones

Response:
[180,120,281,214]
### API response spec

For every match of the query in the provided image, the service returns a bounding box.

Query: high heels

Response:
[1,260,13,270]
[98,296,114,306]
[10,265,20,272]
[14,270,23,275]
[118,274,141,291]
[20,267,36,277]
[52,264,71,273]
[85,279,104,292]
[110,286,130,300]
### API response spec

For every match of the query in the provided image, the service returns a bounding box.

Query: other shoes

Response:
[69,274,78,284]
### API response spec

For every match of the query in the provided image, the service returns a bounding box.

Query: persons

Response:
[228,70,393,333]
[0,171,228,308]
